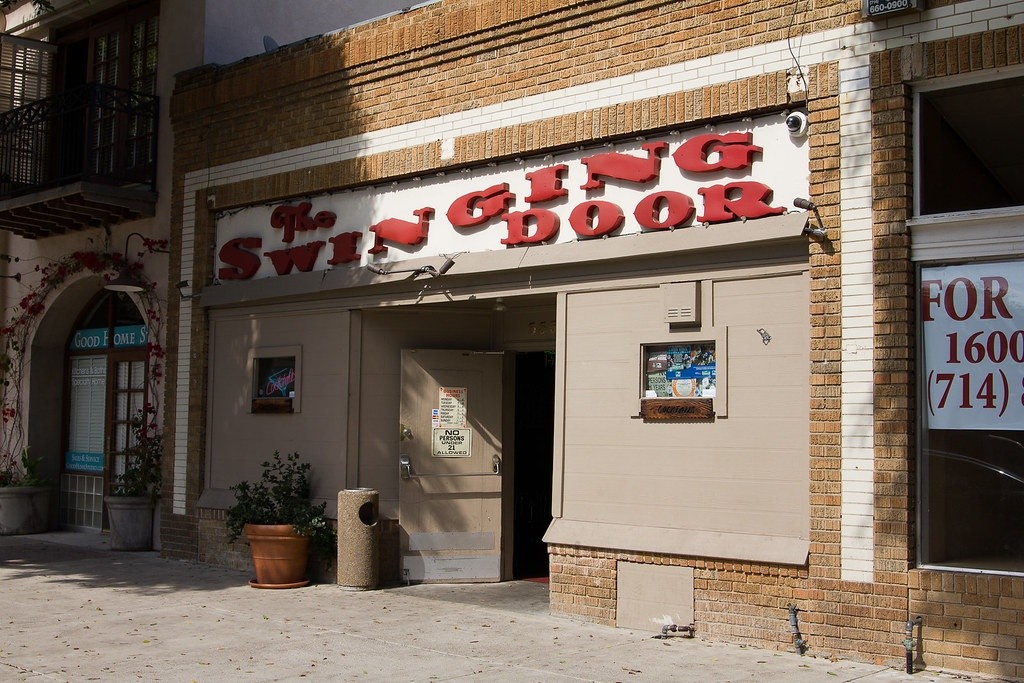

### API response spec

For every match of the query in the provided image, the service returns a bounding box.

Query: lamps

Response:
[104,232,172,293]
[174,279,202,301]
[794,197,828,236]
[491,297,509,313]
[366,258,456,280]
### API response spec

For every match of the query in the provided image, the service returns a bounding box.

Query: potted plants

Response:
[226,447,335,589]
[103,438,161,552]
[0,442,56,536]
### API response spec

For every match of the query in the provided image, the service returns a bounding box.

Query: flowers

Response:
[6,236,168,449]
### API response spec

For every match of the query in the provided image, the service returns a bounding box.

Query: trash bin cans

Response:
[337,488,380,591]
